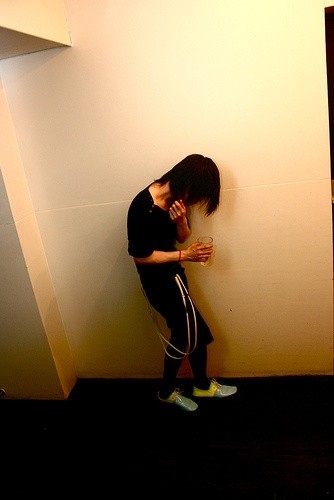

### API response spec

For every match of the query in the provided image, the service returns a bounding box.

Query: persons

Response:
[126,154,237,411]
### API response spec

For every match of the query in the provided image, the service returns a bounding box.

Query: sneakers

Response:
[157,388,198,412]
[192,378,237,396]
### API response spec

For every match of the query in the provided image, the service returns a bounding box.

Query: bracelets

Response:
[178,249,182,262]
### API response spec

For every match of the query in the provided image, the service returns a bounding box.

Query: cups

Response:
[198,236,213,266]
